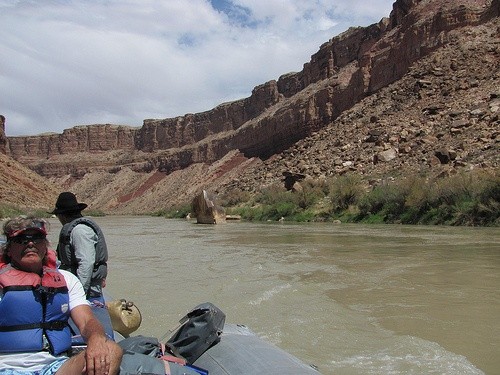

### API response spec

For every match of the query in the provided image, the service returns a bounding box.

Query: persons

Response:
[0,216,124,375]
[47,192,108,299]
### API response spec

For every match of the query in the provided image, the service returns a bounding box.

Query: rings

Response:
[100,360,106,362]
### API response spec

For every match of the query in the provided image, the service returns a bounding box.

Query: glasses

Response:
[11,233,45,244]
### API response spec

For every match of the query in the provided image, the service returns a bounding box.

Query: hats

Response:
[46,191,88,214]
[4,213,47,239]
